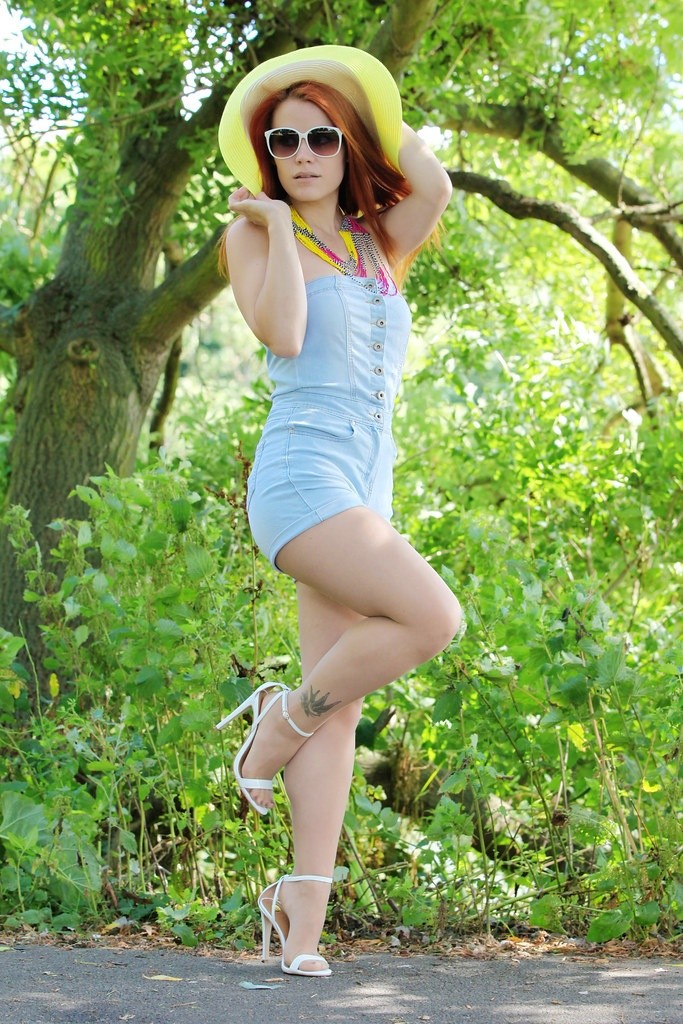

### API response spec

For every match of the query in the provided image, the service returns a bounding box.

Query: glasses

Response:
[264,126,343,160]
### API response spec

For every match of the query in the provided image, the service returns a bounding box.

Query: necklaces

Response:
[283,202,399,299]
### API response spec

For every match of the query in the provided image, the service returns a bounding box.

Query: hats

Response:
[219,44,404,218]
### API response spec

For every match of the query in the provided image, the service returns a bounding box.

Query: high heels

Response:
[258,874,333,976]
[216,682,314,816]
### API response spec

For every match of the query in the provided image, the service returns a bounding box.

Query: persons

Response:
[213,44,467,978]
